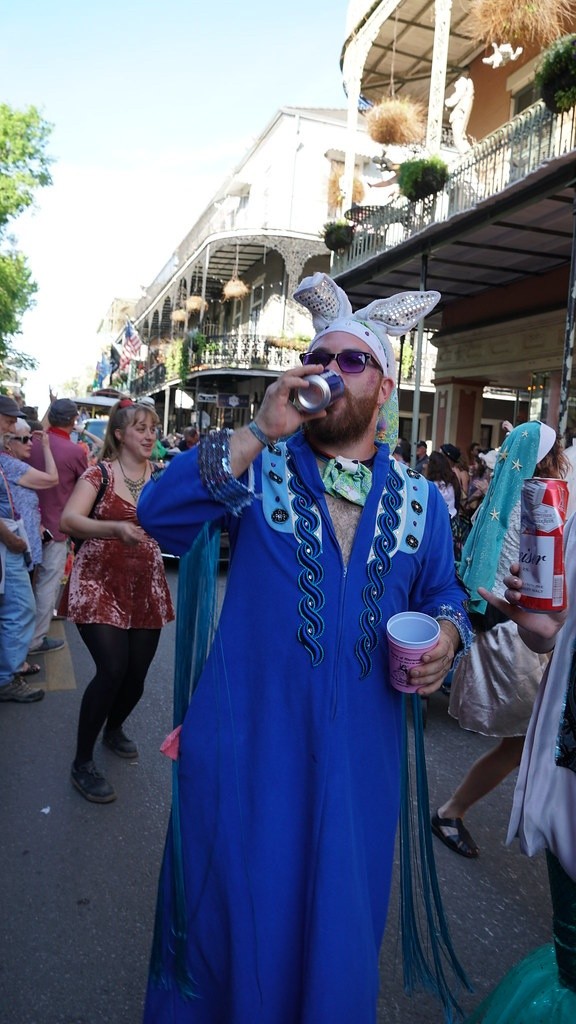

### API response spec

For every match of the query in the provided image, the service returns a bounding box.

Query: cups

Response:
[385,611,441,694]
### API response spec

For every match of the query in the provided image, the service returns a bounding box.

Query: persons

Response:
[390,421,572,857]
[136,331,473,1024]
[0,385,104,704]
[444,77,474,165]
[476,511,576,994]
[151,423,209,460]
[59,398,176,803]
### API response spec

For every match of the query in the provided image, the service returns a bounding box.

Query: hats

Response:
[51,398,79,420]
[479,450,497,470]
[415,440,427,449]
[393,438,411,456]
[0,395,28,419]
[441,444,461,464]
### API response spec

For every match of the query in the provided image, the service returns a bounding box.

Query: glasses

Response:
[299,352,383,373]
[11,436,33,444]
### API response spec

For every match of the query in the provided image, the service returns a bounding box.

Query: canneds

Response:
[517,477,569,613]
[293,370,345,413]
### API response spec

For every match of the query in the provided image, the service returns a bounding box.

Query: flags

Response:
[120,318,141,370]
[108,345,122,386]
[91,354,110,388]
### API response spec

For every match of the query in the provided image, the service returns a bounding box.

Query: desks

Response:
[345,205,406,251]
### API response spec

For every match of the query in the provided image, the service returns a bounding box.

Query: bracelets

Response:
[82,429,85,434]
[249,421,270,446]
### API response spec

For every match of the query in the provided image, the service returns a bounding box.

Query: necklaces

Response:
[117,457,147,502]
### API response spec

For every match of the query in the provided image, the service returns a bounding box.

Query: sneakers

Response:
[72,759,116,803]
[0,675,44,702]
[103,726,138,758]
[28,636,65,655]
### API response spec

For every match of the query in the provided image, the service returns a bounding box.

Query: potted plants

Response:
[319,222,354,252]
[531,35,576,114]
[395,157,449,203]
[179,330,207,384]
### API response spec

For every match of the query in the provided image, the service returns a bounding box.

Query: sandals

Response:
[17,662,41,675]
[431,805,481,858]
